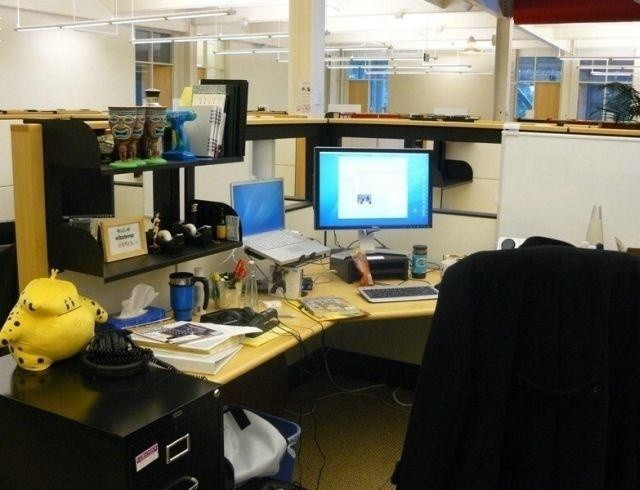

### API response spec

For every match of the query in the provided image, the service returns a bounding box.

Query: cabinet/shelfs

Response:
[22,117,244,285]
[0,352,224,489]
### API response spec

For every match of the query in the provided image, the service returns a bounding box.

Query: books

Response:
[171,83,239,159]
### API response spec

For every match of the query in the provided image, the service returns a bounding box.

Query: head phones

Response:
[81,332,150,377]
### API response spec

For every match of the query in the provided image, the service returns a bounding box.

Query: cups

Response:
[210,271,242,310]
[169,271,209,322]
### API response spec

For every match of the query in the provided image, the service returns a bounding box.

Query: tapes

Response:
[183,223,197,235]
[157,228,173,242]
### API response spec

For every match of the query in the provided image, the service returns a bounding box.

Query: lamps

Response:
[325,1,497,76]
[515,25,640,76]
[15,2,289,64]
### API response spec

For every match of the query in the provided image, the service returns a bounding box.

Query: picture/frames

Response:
[98,215,150,263]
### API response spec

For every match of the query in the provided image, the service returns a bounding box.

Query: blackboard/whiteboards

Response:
[494,129,640,253]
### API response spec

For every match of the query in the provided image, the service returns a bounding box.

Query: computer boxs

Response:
[328,247,409,284]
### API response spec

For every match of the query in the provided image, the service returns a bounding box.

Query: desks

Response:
[127,256,442,386]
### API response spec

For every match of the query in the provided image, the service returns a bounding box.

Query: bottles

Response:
[582,203,603,246]
[142,88,161,108]
[244,261,259,312]
[216,207,226,241]
[192,266,208,317]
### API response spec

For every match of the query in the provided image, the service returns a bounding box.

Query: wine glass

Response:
[107,106,167,169]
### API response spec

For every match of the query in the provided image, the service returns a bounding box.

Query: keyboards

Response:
[357,284,440,303]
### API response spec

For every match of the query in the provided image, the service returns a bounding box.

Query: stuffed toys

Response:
[0,269,108,372]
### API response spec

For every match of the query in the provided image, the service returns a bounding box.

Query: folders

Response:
[149,344,243,377]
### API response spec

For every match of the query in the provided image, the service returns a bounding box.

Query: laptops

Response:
[230,177,331,265]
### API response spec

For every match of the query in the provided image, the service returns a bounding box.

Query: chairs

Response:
[415,137,475,210]
[390,241,640,489]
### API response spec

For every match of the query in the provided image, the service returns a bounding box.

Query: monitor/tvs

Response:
[313,147,433,260]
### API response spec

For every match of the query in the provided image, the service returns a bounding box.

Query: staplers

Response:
[244,307,281,339]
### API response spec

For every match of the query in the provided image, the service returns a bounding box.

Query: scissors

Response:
[208,270,220,297]
[229,259,247,288]
